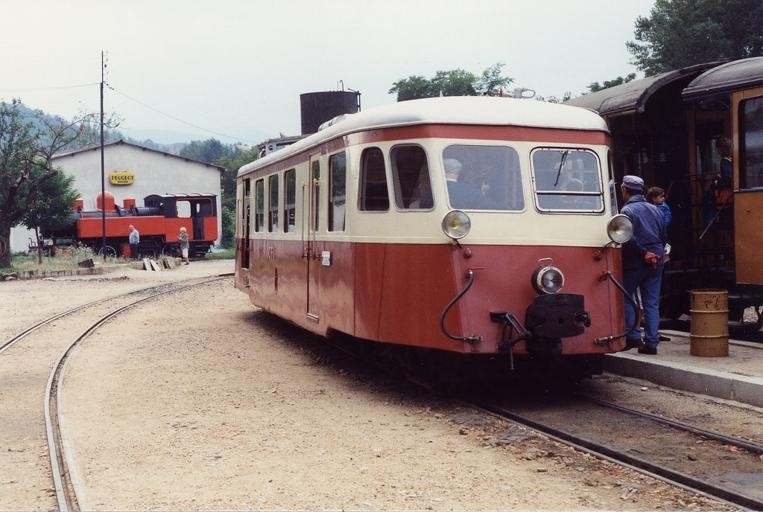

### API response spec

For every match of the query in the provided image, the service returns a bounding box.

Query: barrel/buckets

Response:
[689,290,730,356]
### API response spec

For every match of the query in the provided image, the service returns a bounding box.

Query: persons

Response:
[645,186,673,228]
[560,178,593,210]
[631,184,672,343]
[419,158,500,210]
[714,132,734,186]
[617,174,668,356]
[127,224,140,261]
[479,169,506,209]
[408,161,431,210]
[176,227,190,265]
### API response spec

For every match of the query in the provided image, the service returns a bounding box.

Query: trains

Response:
[558,54,763,340]
[227,87,637,393]
[26,189,219,262]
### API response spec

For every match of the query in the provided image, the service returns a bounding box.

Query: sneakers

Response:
[626,337,644,349]
[637,343,658,355]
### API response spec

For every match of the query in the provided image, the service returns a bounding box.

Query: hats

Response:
[618,173,645,192]
